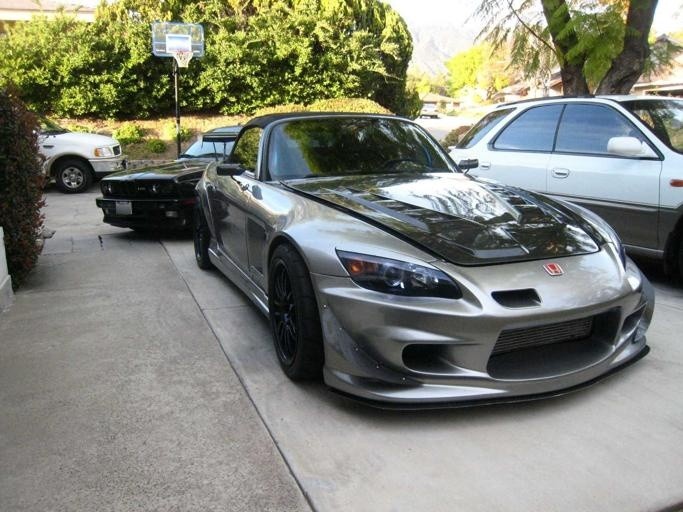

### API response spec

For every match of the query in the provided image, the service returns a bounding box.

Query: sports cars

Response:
[191,111,656,414]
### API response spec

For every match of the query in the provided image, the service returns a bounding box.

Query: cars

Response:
[420,103,439,119]
[32,114,129,195]
[451,93,683,291]
[95,124,243,240]
